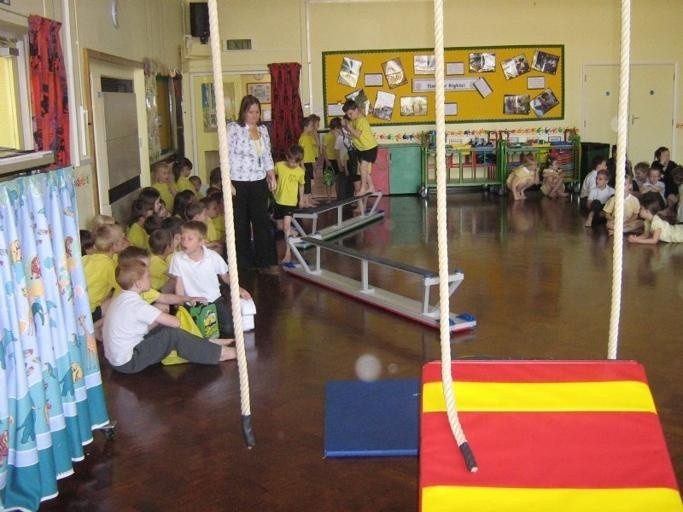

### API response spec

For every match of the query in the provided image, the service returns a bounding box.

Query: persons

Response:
[206,188,220,197]
[168,221,251,339]
[335,125,356,196]
[148,228,175,292]
[298,117,320,210]
[184,202,223,258]
[343,98,379,196]
[321,116,341,204]
[267,144,305,264]
[200,197,220,240]
[80,225,125,344]
[171,191,194,222]
[188,176,206,203]
[507,154,539,202]
[143,212,164,235]
[540,155,571,199]
[157,199,171,219]
[210,193,226,239]
[171,158,200,203]
[516,56,530,75]
[580,143,682,244]
[162,216,183,251]
[210,166,222,188]
[118,246,208,333]
[538,92,556,110]
[139,188,161,218]
[127,199,154,252]
[102,261,238,374]
[151,162,178,211]
[309,115,322,181]
[536,55,557,75]
[91,214,115,231]
[225,95,282,277]
[80,229,98,258]
[182,190,195,203]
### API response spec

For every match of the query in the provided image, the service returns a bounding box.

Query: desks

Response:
[420,359,683,512]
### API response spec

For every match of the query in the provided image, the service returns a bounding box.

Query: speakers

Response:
[190,2,210,44]
[581,143,610,180]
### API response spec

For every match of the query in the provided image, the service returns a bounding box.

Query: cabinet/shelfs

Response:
[504,136,580,193]
[370,142,425,196]
[581,142,610,189]
[425,132,504,197]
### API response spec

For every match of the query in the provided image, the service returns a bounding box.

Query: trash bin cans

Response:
[581,142,610,189]
